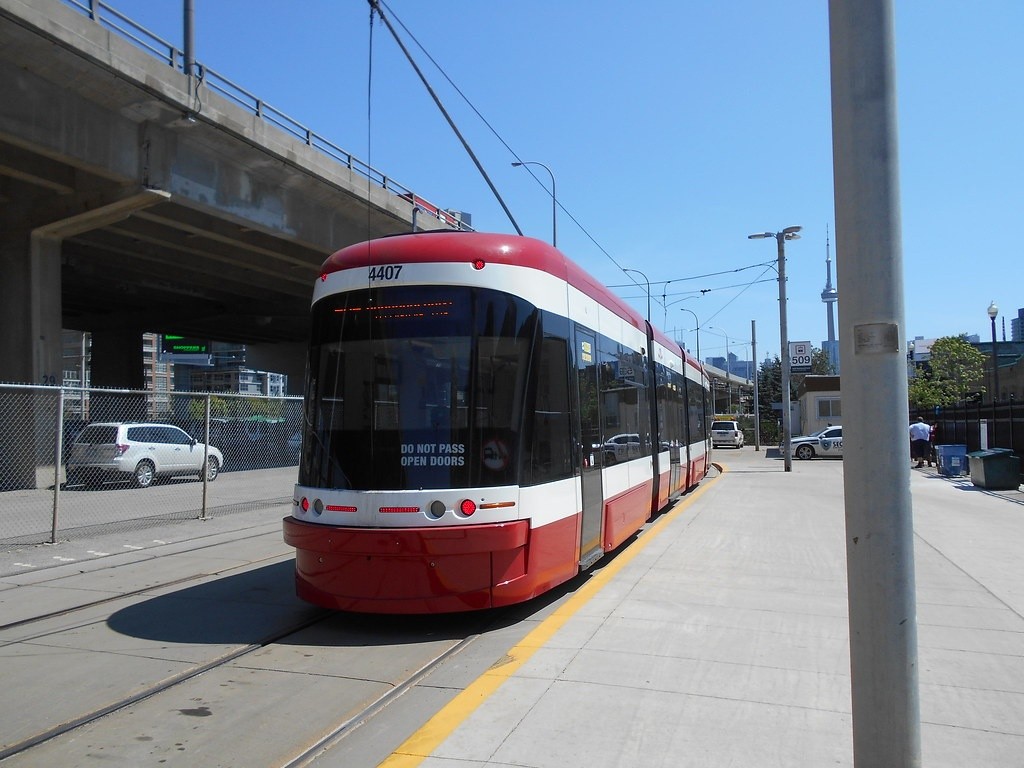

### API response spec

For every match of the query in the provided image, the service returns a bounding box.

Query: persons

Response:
[909,415,937,468]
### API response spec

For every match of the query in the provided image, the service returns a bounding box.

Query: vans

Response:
[711,420,745,449]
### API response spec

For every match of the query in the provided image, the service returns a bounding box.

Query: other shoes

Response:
[915,462,924,468]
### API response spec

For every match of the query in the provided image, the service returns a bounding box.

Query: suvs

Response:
[68,420,224,490]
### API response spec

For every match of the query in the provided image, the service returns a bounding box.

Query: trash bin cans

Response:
[965,447,1020,489]
[934,444,966,475]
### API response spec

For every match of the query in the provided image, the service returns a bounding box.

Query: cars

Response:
[779,425,843,460]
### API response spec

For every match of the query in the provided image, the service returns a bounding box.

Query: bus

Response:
[282,206,712,618]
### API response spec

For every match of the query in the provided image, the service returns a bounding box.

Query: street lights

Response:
[681,309,700,361]
[512,161,556,248]
[710,326,732,414]
[987,300,999,448]
[748,225,803,474]
[623,269,650,322]
